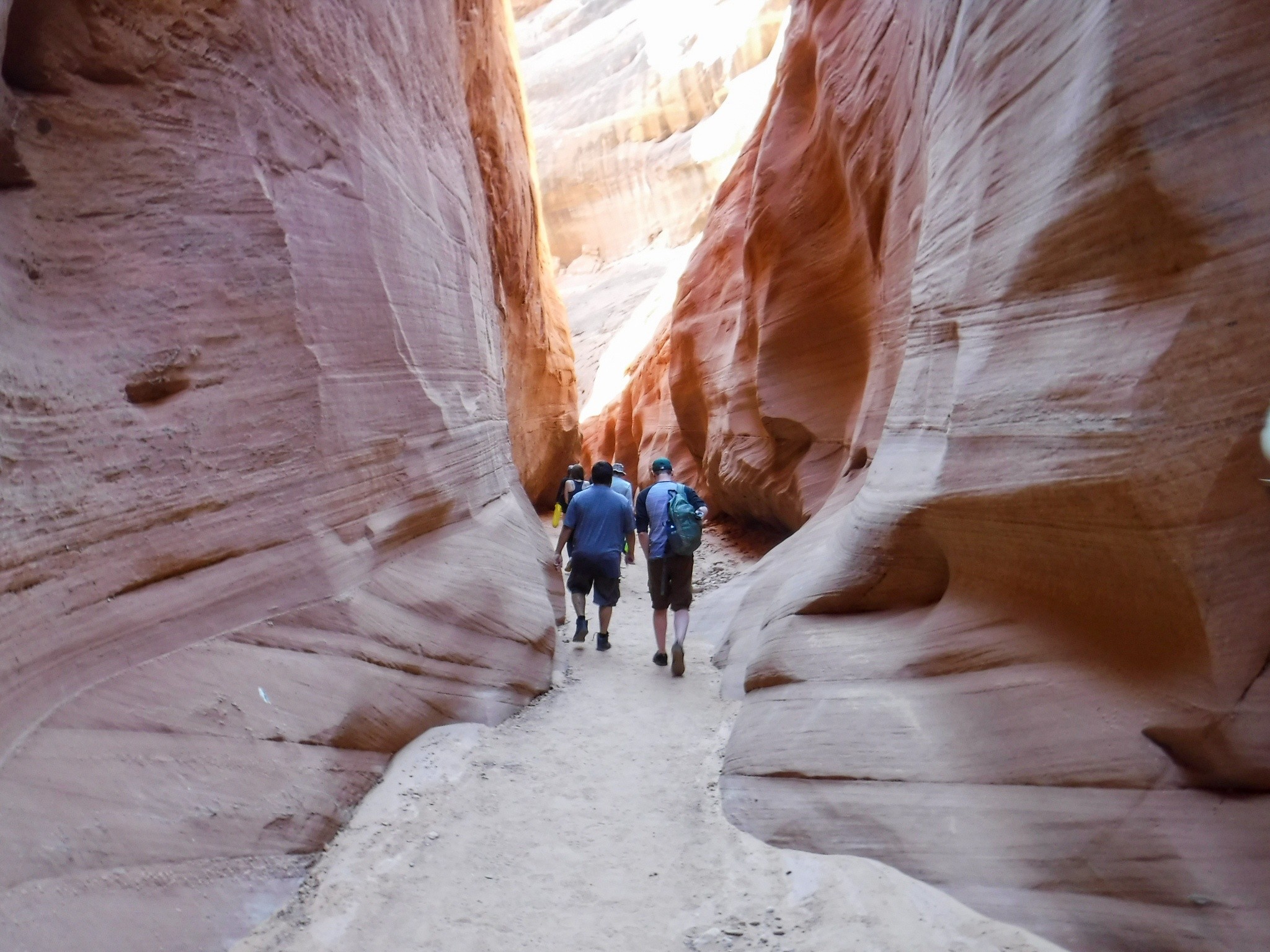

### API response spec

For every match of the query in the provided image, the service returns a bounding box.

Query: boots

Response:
[597,631,610,651]
[573,616,588,642]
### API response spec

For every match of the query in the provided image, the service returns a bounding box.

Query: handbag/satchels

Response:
[552,504,561,528]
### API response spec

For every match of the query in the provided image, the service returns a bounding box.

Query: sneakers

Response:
[671,638,685,676]
[653,650,667,666]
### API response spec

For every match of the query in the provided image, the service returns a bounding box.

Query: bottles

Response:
[697,512,704,525]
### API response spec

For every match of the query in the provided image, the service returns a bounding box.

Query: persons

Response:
[635,458,709,676]
[555,463,633,572]
[552,461,635,652]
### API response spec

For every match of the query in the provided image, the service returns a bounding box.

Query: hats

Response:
[652,457,672,470]
[612,463,626,475]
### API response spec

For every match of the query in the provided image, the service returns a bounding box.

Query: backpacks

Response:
[668,483,704,555]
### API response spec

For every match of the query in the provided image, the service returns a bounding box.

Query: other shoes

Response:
[565,557,573,573]
